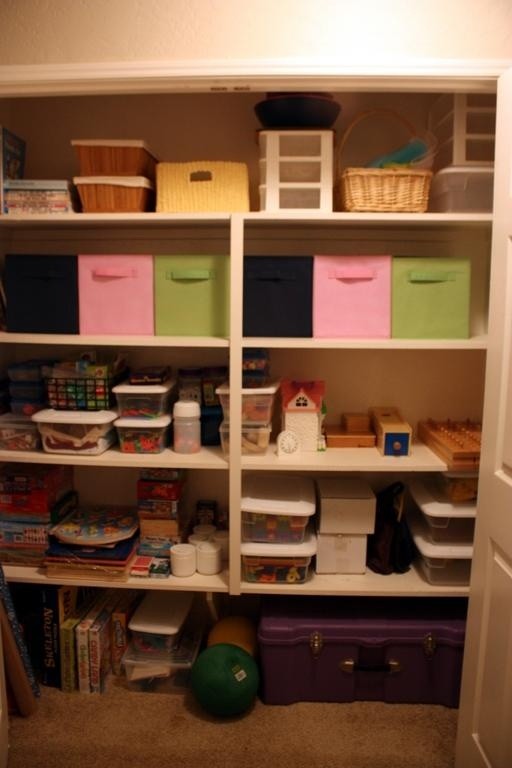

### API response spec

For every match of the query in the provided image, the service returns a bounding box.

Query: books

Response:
[10,581,129,695]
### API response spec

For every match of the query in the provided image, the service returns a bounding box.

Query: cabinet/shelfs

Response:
[0,71,492,610]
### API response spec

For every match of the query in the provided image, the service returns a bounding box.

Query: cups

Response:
[170,524,229,577]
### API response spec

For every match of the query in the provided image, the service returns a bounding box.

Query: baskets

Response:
[333,107,434,212]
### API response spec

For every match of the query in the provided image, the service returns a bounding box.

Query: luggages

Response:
[256,601,466,705]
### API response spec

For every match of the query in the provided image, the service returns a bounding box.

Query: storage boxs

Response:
[31,406,116,456]
[238,475,476,583]
[66,136,161,213]
[112,369,176,454]
[215,378,282,456]
[257,617,466,710]
[119,592,204,695]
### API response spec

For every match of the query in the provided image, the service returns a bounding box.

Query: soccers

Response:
[193,614,260,719]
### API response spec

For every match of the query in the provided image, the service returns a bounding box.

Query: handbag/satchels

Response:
[367,482,419,574]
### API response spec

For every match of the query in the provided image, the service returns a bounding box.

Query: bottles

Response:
[173,400,202,454]
